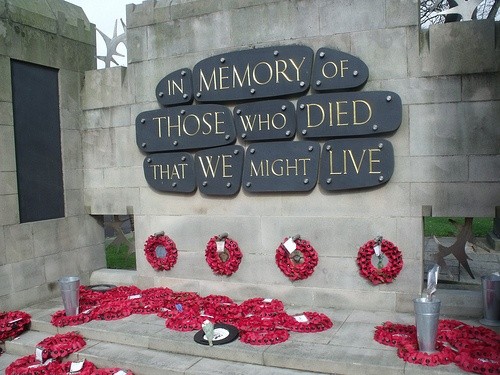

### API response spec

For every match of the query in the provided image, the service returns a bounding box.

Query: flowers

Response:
[0,233,500,375]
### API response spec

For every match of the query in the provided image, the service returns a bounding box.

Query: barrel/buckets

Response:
[59,276,80,316]
[480,275,500,319]
[414,299,440,352]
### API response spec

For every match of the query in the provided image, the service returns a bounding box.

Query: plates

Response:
[193,324,238,344]
